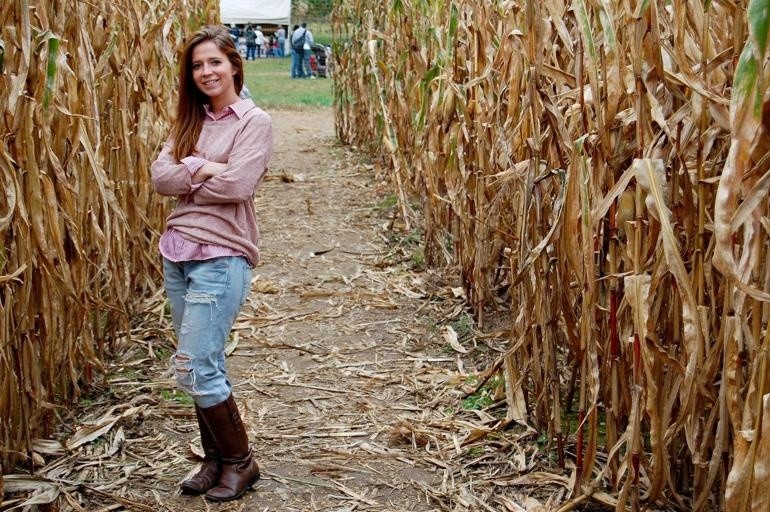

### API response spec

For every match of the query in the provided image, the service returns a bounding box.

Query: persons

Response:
[229,21,317,80]
[150,25,273,502]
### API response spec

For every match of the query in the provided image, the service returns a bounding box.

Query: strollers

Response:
[310,43,335,79]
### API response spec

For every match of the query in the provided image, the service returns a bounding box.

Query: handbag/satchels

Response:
[293,37,306,55]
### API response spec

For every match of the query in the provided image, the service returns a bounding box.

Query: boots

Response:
[196,390,260,501]
[180,398,223,496]
